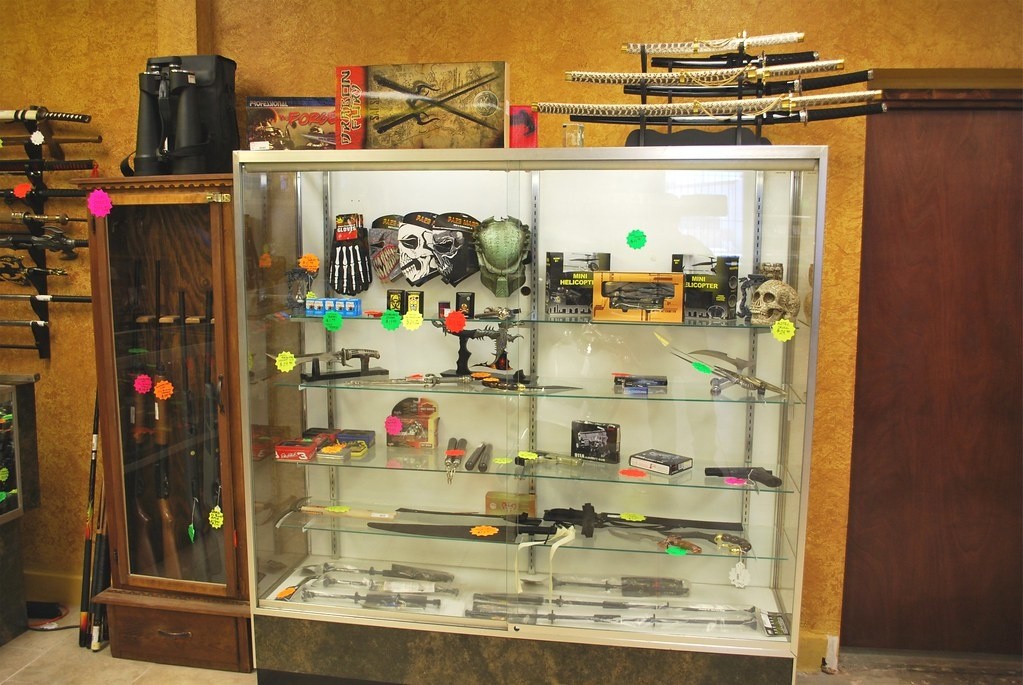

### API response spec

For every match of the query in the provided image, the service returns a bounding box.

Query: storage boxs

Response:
[276,428,377,462]
[628,449,692,475]
[245,97,336,150]
[672,255,739,320]
[334,61,510,149]
[592,271,684,325]
[486,491,536,519]
[386,398,440,447]
[546,252,611,315]
[571,420,620,465]
[612,375,668,387]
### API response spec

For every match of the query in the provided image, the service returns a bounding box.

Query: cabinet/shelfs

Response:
[234,146,830,685]
[72,174,253,674]
